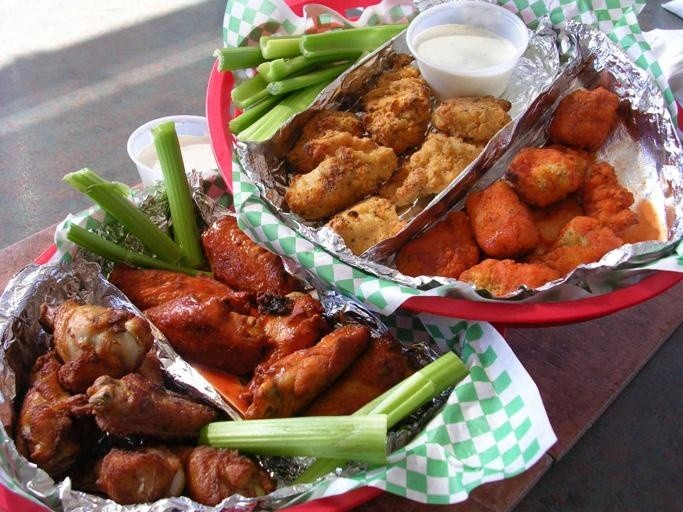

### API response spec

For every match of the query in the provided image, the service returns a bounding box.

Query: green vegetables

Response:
[58,120,211,280]
[203,350,467,486]
[211,26,408,142]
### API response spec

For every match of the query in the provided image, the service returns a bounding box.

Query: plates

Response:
[1,172,485,512]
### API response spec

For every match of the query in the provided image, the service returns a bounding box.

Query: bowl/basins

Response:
[404,0,530,103]
[125,113,220,181]
